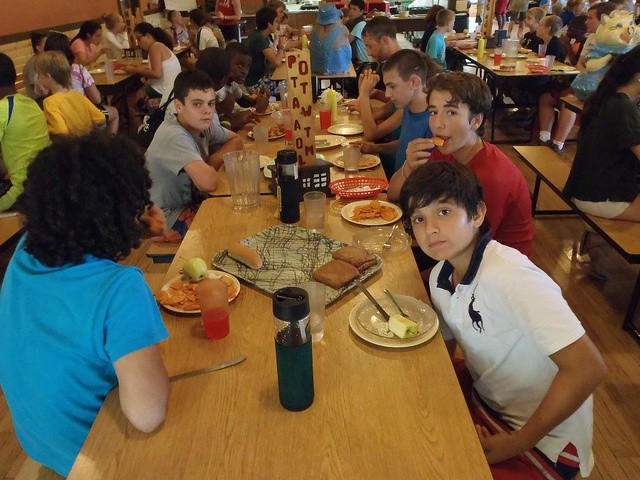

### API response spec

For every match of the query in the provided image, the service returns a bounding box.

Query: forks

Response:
[383,224,398,249]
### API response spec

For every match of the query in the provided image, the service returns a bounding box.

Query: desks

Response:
[386,13,428,32]
[288,8,318,27]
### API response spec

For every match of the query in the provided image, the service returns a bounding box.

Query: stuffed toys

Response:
[570,9,640,102]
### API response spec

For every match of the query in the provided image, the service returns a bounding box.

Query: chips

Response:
[349,200,397,222]
[157,280,234,311]
[432,137,444,146]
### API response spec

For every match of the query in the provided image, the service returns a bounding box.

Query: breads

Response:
[314,260,359,289]
[228,243,262,269]
[332,244,377,272]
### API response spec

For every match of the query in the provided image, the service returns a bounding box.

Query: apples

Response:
[184,258,207,282]
[388,314,418,339]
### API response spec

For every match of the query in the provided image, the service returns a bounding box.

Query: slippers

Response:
[580,230,590,255]
[573,258,610,280]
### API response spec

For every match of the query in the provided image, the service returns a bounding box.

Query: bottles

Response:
[327,85,337,121]
[477,36,484,61]
[272,286,313,412]
[276,150,300,224]
[301,34,308,49]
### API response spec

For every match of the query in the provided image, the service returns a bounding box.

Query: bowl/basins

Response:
[455,40,477,50]
[271,110,316,131]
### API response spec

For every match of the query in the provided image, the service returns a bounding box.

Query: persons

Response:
[205,13,227,49]
[70,20,110,65]
[309,4,351,74]
[158,47,263,154]
[566,14,592,56]
[211,0,242,42]
[425,10,456,73]
[280,11,301,38]
[507,1,528,39]
[33,50,106,142]
[519,14,563,131]
[400,161,605,479]
[559,0,584,27]
[559,46,640,280]
[260,1,304,54]
[145,67,244,241]
[341,0,380,98]
[125,22,184,128]
[214,40,270,121]
[421,4,467,72]
[24,28,49,99]
[1,52,52,211]
[549,1,566,17]
[350,48,434,181]
[167,10,190,47]
[0,135,169,480]
[343,15,440,114]
[356,52,439,142]
[102,13,131,58]
[493,0,509,36]
[387,72,535,285]
[243,9,286,101]
[31,34,120,139]
[492,8,545,121]
[528,3,616,155]
[178,9,223,72]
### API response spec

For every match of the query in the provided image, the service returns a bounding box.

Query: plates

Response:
[327,124,365,135]
[315,135,346,149]
[492,64,518,71]
[263,160,275,179]
[157,270,241,314]
[330,150,381,170]
[114,70,128,74]
[352,226,413,256]
[247,127,287,140]
[260,155,269,169]
[489,53,528,58]
[348,294,440,349]
[88,69,105,74]
[212,224,383,306]
[341,200,402,226]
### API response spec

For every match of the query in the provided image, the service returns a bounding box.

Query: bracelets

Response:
[402,159,411,180]
[277,45,286,51]
[510,431,526,461]
[223,15,227,23]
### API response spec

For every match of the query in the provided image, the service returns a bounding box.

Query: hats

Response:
[315,2,343,25]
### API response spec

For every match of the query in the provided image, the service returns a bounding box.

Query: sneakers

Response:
[526,136,565,156]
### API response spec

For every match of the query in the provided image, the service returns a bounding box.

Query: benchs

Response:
[512,144,640,343]
[0,211,24,245]
[146,240,181,263]
[0,212,30,249]
[138,272,165,297]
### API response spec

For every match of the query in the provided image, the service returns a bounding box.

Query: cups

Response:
[343,144,361,176]
[303,191,327,234]
[223,150,261,210]
[494,48,502,66]
[320,109,331,129]
[297,282,326,343]
[253,125,270,154]
[196,279,230,340]
[538,44,547,58]
[546,55,555,67]
[282,117,293,146]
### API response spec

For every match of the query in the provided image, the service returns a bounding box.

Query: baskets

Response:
[329,177,388,199]
[527,65,548,74]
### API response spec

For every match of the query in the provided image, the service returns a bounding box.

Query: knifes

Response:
[168,354,248,382]
